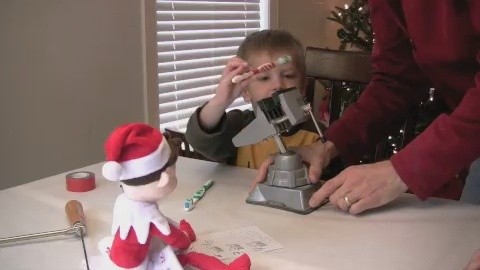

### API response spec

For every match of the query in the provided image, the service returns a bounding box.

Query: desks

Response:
[0,155,479,270]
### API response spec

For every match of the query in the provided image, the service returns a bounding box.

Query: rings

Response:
[344,195,351,209]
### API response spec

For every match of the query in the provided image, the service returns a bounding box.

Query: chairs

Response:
[302,46,422,165]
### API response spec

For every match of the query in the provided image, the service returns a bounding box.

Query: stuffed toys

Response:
[97,123,253,270]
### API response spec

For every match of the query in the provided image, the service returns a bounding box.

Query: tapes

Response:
[66,171,95,192]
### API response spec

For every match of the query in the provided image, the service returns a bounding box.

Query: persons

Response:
[184,29,344,181]
[248,0,480,219]
